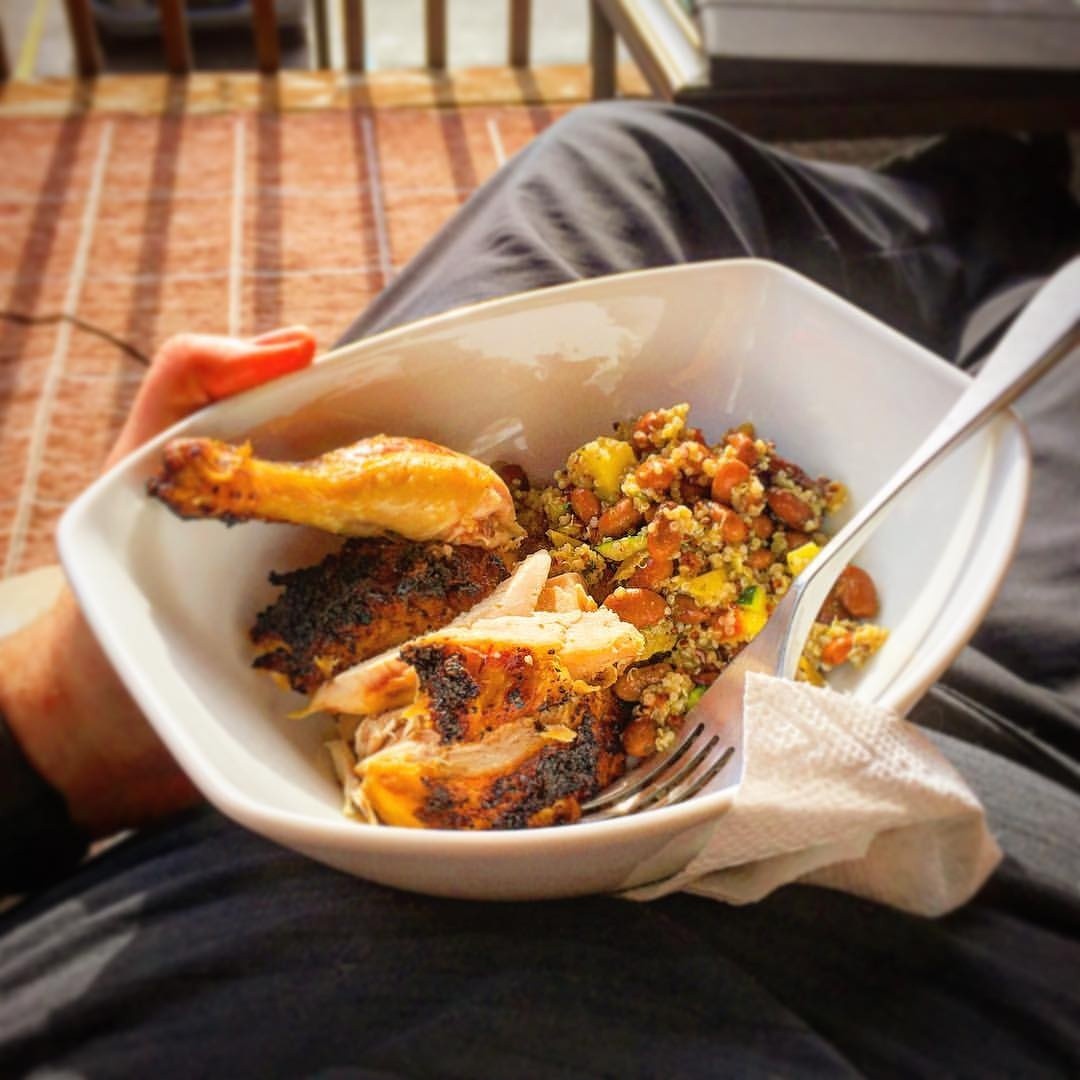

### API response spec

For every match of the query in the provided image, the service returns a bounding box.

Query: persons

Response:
[0,95,1080,1080]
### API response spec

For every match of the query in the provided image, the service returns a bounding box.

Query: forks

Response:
[580,254,1080,831]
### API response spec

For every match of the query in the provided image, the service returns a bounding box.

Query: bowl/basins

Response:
[55,251,1033,904]
